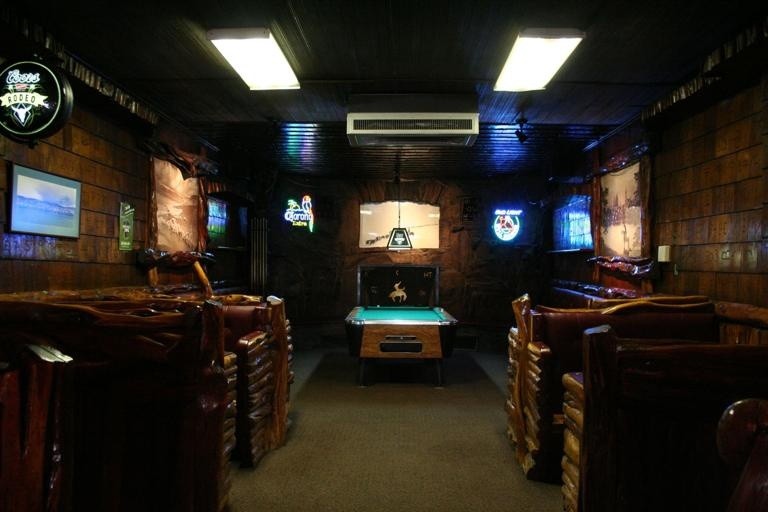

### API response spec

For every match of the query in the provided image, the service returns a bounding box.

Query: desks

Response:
[344,304,459,388]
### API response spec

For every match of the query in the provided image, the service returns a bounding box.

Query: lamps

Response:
[492,25,586,92]
[205,21,300,91]
[387,155,412,251]
[516,119,529,144]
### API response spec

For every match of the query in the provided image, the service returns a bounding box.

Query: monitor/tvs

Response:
[4,163,82,240]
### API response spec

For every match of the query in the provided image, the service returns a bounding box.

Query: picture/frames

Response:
[585,135,660,279]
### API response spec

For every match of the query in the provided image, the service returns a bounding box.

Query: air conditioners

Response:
[346,113,479,147]
